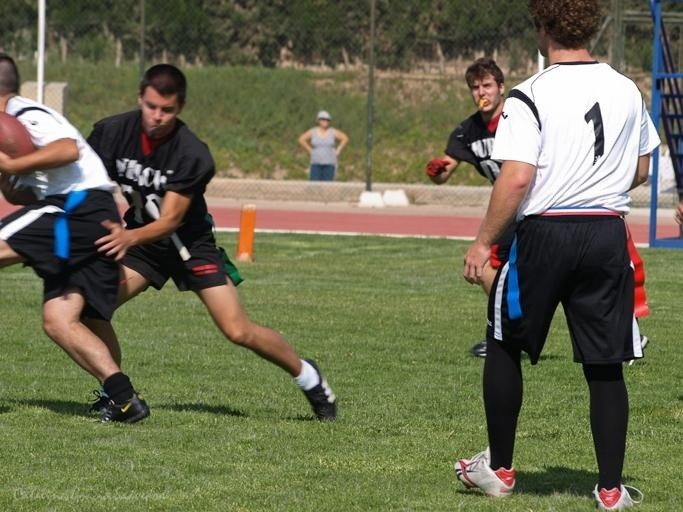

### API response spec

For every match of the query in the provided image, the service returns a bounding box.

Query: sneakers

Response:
[93,391,151,426]
[591,481,644,512]
[88,389,115,417]
[469,340,489,359]
[299,356,339,422]
[454,445,517,500]
[624,333,651,368]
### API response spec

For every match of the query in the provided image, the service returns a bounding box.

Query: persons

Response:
[448,1,660,509]
[293,106,352,181]
[0,52,148,426]
[426,58,515,355]
[77,61,338,419]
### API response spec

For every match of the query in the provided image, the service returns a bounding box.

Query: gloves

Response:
[424,159,452,178]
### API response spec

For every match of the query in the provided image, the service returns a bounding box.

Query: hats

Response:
[316,110,332,122]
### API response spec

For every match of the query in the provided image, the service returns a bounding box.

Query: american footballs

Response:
[0,111,35,159]
